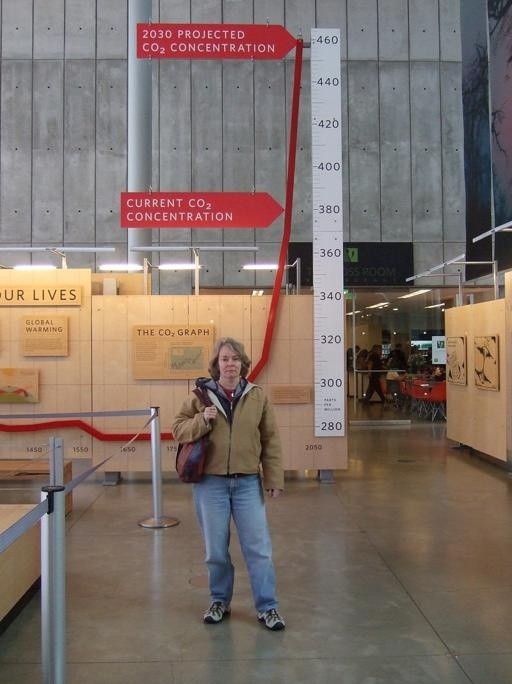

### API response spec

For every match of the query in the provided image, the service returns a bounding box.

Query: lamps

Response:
[240,257,302,295]
[96,261,203,272]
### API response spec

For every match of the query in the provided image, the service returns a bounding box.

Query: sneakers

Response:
[257,609,286,631]
[203,599,231,624]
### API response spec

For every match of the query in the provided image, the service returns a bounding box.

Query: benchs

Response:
[0,460,72,520]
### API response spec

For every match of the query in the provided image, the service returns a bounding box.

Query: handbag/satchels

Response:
[175,440,211,483]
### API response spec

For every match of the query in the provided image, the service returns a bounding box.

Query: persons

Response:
[347,343,432,404]
[171,339,285,629]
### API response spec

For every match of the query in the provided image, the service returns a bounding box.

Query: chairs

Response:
[398,378,447,422]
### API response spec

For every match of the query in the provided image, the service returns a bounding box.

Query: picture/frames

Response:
[444,336,502,393]
